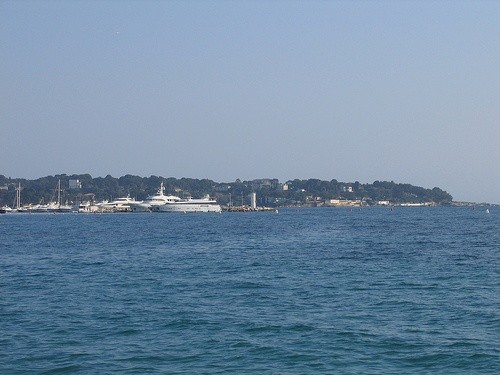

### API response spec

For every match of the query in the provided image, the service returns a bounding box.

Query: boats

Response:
[19,204,37,213]
[148,194,222,213]
[99,196,142,211]
[78,201,107,212]
[1,204,12,212]
[126,181,180,212]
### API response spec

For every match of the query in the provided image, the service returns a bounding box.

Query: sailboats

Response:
[37,179,72,213]
